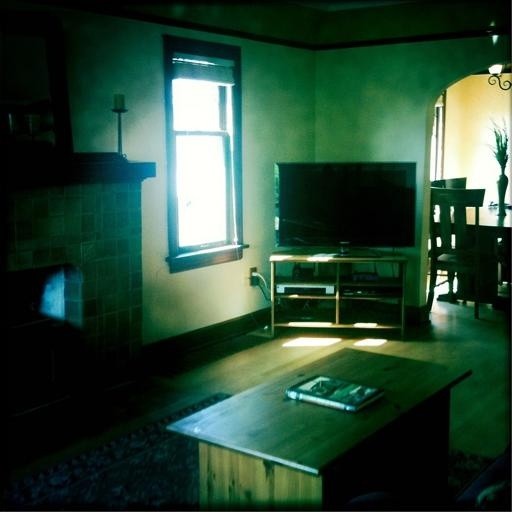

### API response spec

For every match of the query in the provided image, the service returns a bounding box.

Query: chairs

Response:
[426,180,484,319]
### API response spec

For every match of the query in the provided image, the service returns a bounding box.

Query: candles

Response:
[114,94,125,109]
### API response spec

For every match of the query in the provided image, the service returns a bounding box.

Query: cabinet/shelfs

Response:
[268,246,409,337]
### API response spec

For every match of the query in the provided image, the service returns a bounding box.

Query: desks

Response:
[166,348,474,510]
[428,198,512,310]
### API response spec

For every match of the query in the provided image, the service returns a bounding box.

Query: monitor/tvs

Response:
[272,160,418,257]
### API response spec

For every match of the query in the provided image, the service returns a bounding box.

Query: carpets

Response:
[4,392,495,509]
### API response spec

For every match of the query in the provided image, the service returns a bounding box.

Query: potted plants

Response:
[484,117,508,215]
